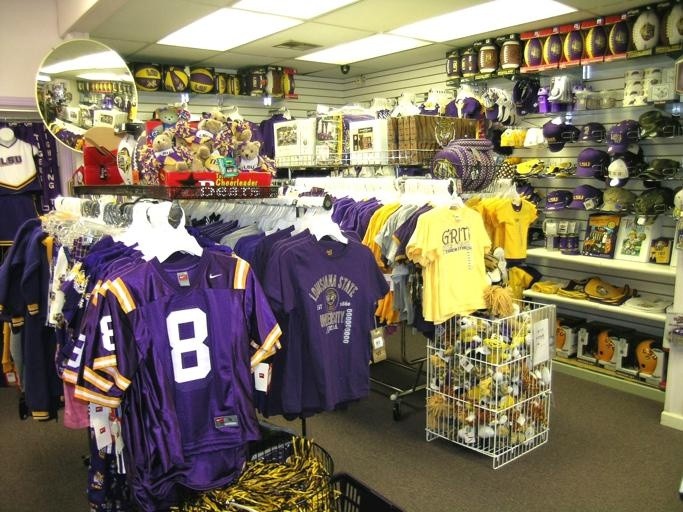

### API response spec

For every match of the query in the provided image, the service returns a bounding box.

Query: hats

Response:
[608,144,643,187]
[545,190,573,210]
[543,122,568,152]
[568,185,602,209]
[639,159,680,181]
[607,120,638,153]
[513,78,540,116]
[577,148,606,177]
[639,111,664,138]
[635,187,675,226]
[482,88,520,126]
[601,187,637,212]
[517,183,538,203]
[579,122,606,143]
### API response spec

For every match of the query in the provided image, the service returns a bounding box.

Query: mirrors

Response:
[35,39,138,153]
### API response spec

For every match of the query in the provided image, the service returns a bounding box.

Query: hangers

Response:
[295,175,464,209]
[468,178,525,205]
[39,193,205,267]
[172,198,349,246]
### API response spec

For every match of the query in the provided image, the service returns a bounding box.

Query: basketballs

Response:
[133,64,162,91]
[162,65,189,92]
[189,68,214,94]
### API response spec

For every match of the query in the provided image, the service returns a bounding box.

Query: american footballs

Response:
[214,66,295,96]
[446,2,683,78]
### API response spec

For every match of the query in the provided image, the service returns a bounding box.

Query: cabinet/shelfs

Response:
[446,0,679,405]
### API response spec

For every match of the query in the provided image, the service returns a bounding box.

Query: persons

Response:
[1,127,44,266]
